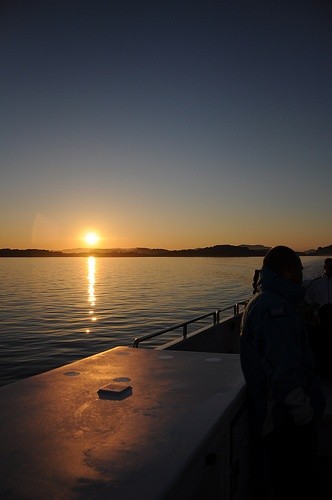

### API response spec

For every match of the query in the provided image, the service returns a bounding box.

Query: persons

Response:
[305,259,332,322]
[239,247,327,497]
